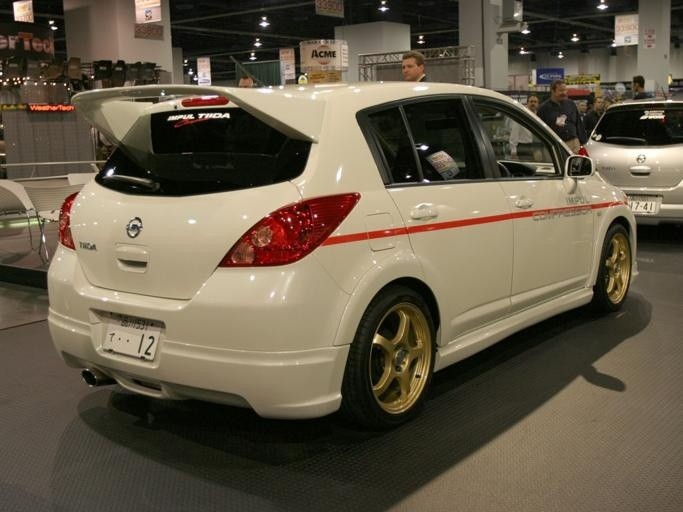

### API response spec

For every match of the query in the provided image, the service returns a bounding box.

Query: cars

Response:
[45,80,646,439]
[581,99,683,230]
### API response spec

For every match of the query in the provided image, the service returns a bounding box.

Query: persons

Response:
[633,76,654,99]
[402,51,432,81]
[527,80,603,154]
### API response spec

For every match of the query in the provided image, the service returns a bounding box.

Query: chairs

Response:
[0,172,98,263]
